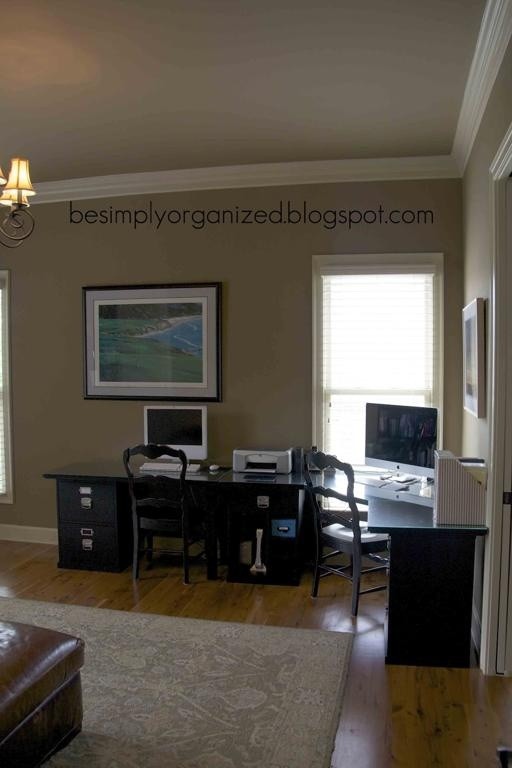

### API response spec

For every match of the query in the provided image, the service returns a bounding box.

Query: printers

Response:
[232,447,294,474]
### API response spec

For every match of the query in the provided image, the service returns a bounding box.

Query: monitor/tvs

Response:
[144,405,207,463]
[365,403,440,478]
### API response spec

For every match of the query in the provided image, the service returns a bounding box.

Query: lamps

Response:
[0,156,37,249]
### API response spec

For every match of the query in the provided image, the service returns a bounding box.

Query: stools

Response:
[0,617,86,767]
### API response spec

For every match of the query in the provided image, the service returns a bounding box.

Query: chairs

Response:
[122,441,220,586]
[299,448,391,621]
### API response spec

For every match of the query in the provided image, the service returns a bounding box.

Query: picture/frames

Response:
[79,279,226,406]
[459,295,487,423]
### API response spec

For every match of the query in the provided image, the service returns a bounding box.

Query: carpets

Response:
[0,591,355,767]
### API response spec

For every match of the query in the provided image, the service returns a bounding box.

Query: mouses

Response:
[209,464,220,470]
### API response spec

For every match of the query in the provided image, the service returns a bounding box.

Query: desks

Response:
[38,457,488,669]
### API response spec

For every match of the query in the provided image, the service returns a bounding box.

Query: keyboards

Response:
[355,475,409,492]
[139,463,201,473]
